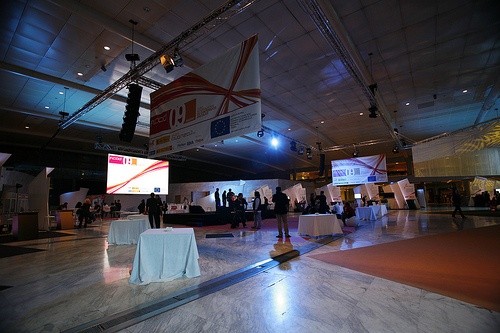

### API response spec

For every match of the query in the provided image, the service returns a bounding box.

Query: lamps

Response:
[298,146,304,155]
[160,54,175,73]
[101,64,107,72]
[257,128,265,138]
[306,148,311,155]
[369,106,379,118]
[290,141,297,151]
[307,155,312,161]
[172,43,184,68]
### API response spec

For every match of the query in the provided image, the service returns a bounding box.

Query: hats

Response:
[150,193,155,196]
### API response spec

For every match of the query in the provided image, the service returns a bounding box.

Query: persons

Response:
[227,189,248,229]
[183,197,194,209]
[474,191,500,217]
[272,186,291,237]
[436,190,467,207]
[331,195,367,225]
[138,199,145,214]
[222,191,227,207]
[251,192,262,229]
[75,199,91,229]
[146,193,168,229]
[88,199,121,224]
[452,190,468,219]
[264,196,268,210]
[288,197,306,213]
[301,190,331,214]
[215,188,220,206]
[56,202,68,210]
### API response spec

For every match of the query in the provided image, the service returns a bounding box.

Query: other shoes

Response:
[275,235,283,238]
[251,226,256,228]
[285,235,292,237]
[256,227,259,229]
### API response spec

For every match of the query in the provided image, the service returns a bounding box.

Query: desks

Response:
[107,219,149,245]
[55,212,74,230]
[298,214,343,236]
[127,215,151,229]
[355,207,376,221]
[11,213,38,238]
[129,228,201,285]
[370,205,388,217]
